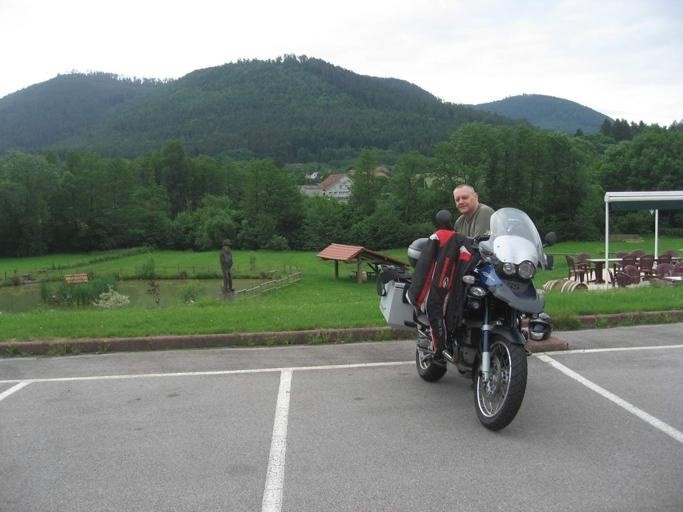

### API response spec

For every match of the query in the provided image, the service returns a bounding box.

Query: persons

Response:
[452,183,507,240]
[220,245,235,293]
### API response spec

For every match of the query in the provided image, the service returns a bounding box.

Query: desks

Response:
[352,270,374,280]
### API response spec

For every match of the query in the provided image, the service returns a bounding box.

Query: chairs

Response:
[565,250,683,288]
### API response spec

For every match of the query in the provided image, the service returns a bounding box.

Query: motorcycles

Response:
[369,206,557,430]
